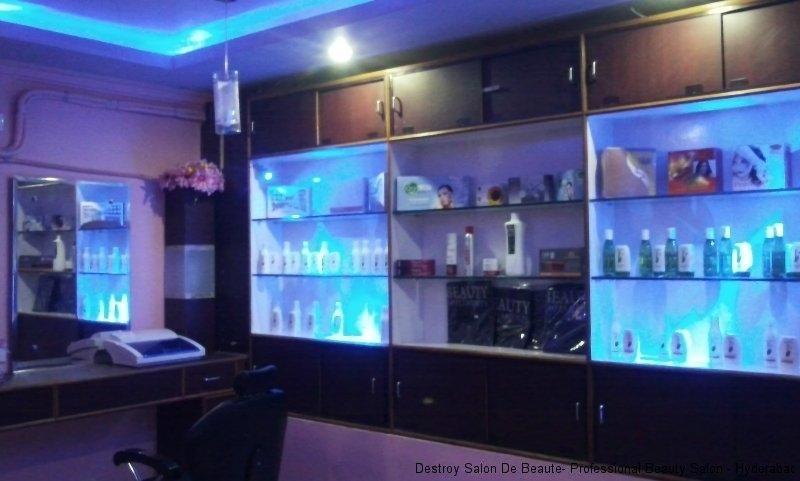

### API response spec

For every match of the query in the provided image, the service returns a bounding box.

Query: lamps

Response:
[212,0,242,137]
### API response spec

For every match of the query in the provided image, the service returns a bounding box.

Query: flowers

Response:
[156,158,225,195]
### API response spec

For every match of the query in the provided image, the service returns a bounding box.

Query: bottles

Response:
[257,236,388,272]
[82,244,129,274]
[719,225,734,275]
[665,226,679,275]
[271,299,389,343]
[602,228,615,275]
[703,227,719,276]
[503,210,526,277]
[611,317,621,350]
[708,315,724,358]
[773,223,787,276]
[762,225,774,277]
[638,228,652,275]
[97,293,129,322]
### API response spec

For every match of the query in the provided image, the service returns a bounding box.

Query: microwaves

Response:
[765,319,777,360]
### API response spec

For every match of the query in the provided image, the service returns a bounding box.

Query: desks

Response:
[0,349,251,433]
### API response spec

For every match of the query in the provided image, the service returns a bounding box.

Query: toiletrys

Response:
[600,222,800,366]
[331,302,343,340]
[445,232,457,277]
[305,302,316,338]
[379,306,390,345]
[82,245,129,322]
[257,238,388,274]
[269,300,282,334]
[464,225,474,276]
[288,299,301,336]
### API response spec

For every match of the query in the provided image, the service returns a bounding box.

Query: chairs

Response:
[112,364,288,480]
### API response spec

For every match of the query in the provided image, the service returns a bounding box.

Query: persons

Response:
[436,183,454,209]
[696,158,713,177]
[732,144,771,194]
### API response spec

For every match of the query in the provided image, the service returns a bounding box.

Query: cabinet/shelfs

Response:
[12,175,131,361]
